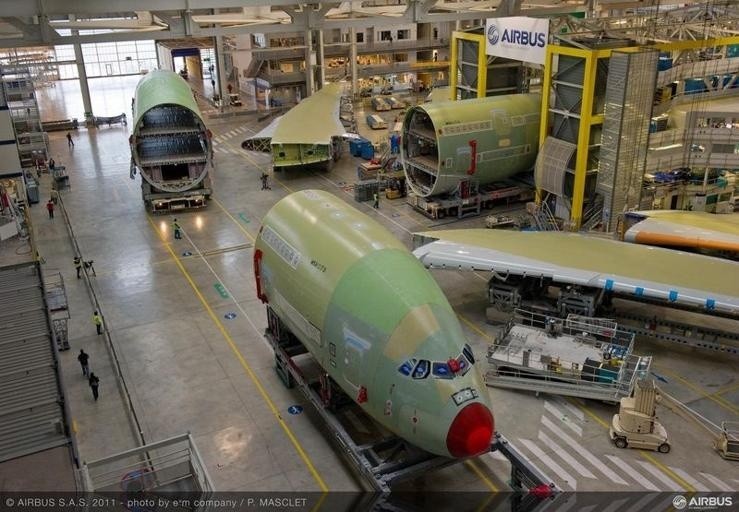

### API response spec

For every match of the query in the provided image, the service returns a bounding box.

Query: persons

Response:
[46,199,55,218]
[87,371,100,402]
[48,157,54,170]
[370,190,380,208]
[72,255,82,279]
[76,349,91,379]
[66,132,75,147]
[48,186,59,204]
[173,218,183,239]
[92,312,104,336]
